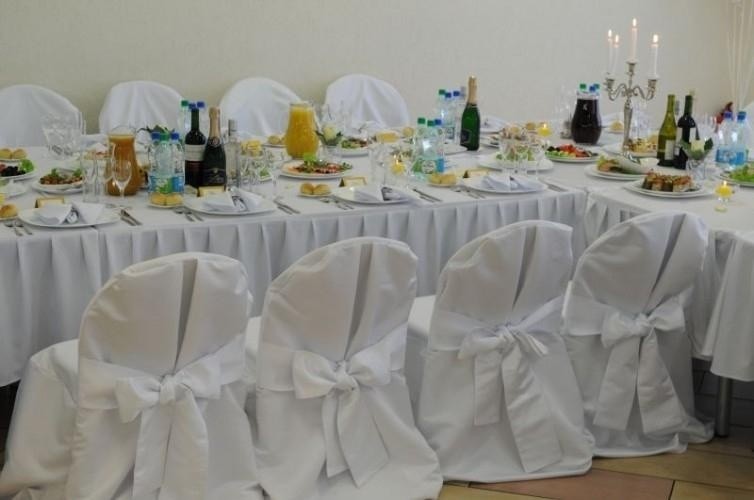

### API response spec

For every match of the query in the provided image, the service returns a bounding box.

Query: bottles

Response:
[460,74,482,150]
[576,81,753,210]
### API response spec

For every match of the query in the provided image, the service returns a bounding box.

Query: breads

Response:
[611,120,624,130]
[300,183,330,194]
[526,121,536,130]
[150,191,182,205]
[269,135,284,144]
[643,172,694,192]
[430,174,457,185]
[0,147,26,159]
[1,204,16,217]
[376,131,397,143]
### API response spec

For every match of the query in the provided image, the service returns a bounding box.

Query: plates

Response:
[2,118,606,228]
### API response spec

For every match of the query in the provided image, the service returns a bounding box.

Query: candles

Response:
[605,14,662,84]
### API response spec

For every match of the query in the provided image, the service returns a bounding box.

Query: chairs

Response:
[214,77,320,127]
[0,82,87,135]
[98,80,197,129]
[321,76,410,125]
[0,246,258,500]
[401,221,597,491]
[248,235,448,500]
[565,206,719,465]
